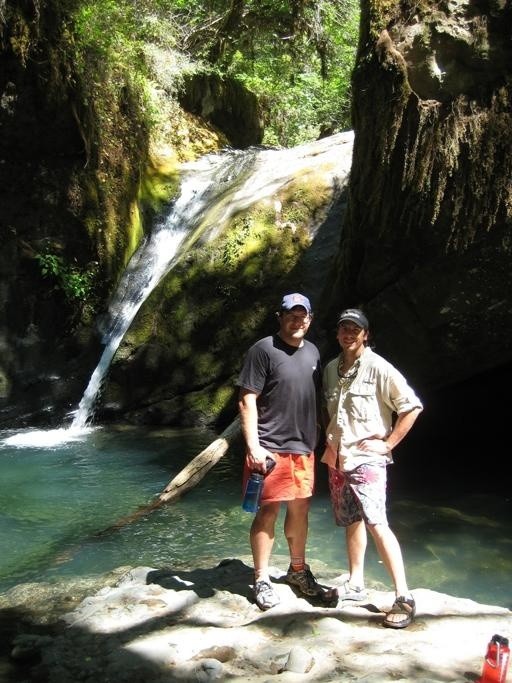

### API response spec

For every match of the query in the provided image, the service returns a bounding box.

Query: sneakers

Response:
[286,563,323,597]
[254,581,279,610]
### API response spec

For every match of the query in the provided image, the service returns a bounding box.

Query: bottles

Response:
[242,469,264,513]
[480,634,511,683]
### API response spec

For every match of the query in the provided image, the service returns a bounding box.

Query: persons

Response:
[321,308,424,627]
[234,293,323,612]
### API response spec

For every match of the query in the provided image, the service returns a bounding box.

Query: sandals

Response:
[384,593,415,627]
[322,580,367,602]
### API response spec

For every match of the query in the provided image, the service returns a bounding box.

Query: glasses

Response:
[337,359,360,378]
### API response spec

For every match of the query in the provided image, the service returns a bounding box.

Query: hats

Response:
[280,293,312,314]
[337,309,369,329]
